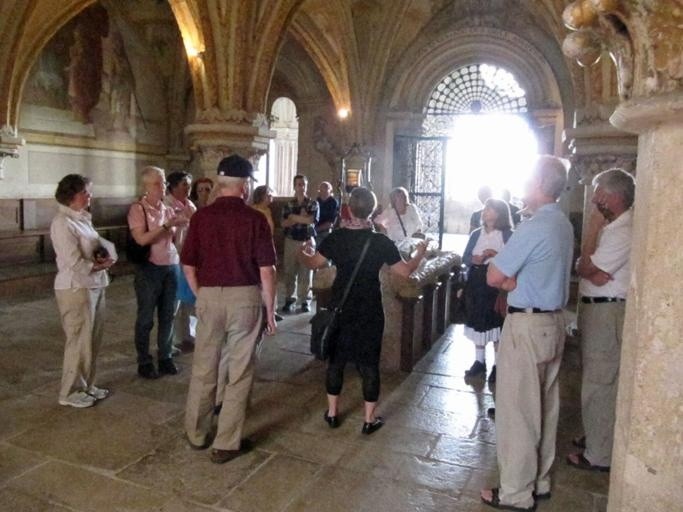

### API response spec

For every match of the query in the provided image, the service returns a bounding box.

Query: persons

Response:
[164,169,197,357]
[460,197,514,383]
[315,180,340,233]
[249,184,287,324]
[339,184,359,225]
[478,154,578,512]
[125,163,181,381]
[467,180,524,234]
[48,172,119,410]
[563,166,639,473]
[193,176,214,208]
[278,173,321,315]
[178,151,280,465]
[298,184,435,437]
[373,185,429,244]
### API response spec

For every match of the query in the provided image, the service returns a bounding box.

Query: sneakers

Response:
[301,301,310,313]
[58,389,96,409]
[281,297,296,312]
[86,385,109,400]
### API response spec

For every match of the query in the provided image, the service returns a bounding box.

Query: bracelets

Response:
[162,224,169,232]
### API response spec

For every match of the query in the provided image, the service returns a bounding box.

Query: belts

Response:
[507,306,562,315]
[580,295,626,304]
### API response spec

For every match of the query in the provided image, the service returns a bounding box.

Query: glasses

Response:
[197,187,212,192]
[146,181,170,188]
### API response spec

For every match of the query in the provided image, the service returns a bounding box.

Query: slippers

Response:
[532,489,551,499]
[479,486,538,512]
[572,433,587,449]
[566,452,611,473]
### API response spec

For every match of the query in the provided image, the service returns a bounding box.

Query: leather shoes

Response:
[210,437,255,465]
[464,359,486,376]
[487,365,496,383]
[185,433,208,451]
[157,352,180,375]
[488,405,496,415]
[324,409,341,428]
[135,360,160,380]
[362,415,385,435]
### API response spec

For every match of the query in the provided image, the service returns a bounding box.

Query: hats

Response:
[216,154,259,184]
